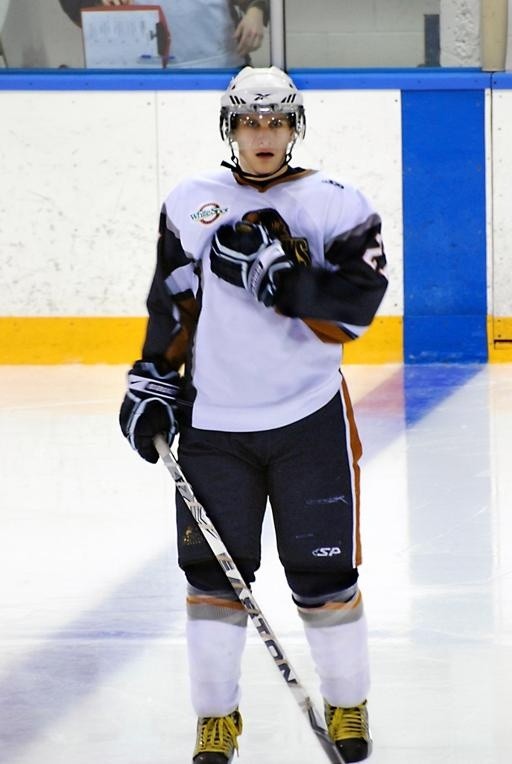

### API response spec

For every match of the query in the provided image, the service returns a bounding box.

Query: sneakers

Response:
[192,710,242,764]
[324,700,372,763]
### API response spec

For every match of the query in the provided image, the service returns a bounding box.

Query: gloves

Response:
[209,220,284,301]
[119,361,183,464]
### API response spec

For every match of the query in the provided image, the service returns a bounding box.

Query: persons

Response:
[115,62,391,764]
[59,0,269,68]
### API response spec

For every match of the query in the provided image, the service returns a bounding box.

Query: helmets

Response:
[219,65,306,142]
